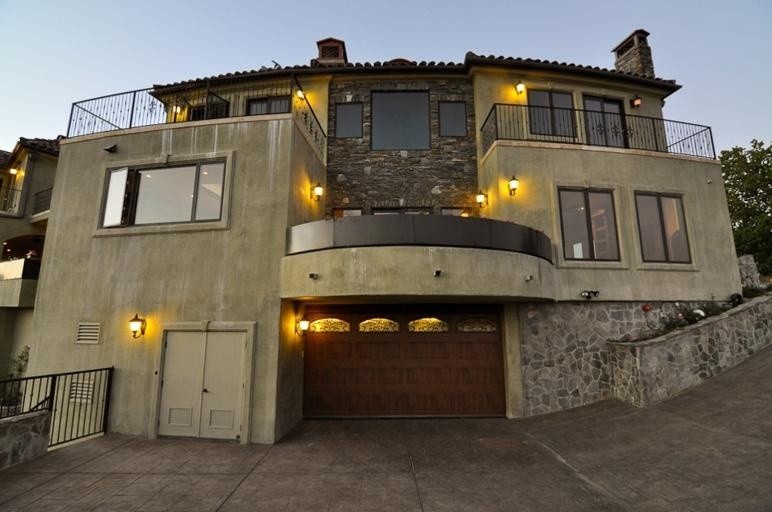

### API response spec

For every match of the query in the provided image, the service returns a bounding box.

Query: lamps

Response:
[514,79,525,95]
[581,290,600,299]
[308,179,326,203]
[128,311,148,341]
[475,190,490,209]
[172,97,183,115]
[506,175,520,196]
[628,94,644,109]
[292,316,312,338]
[297,88,307,100]
